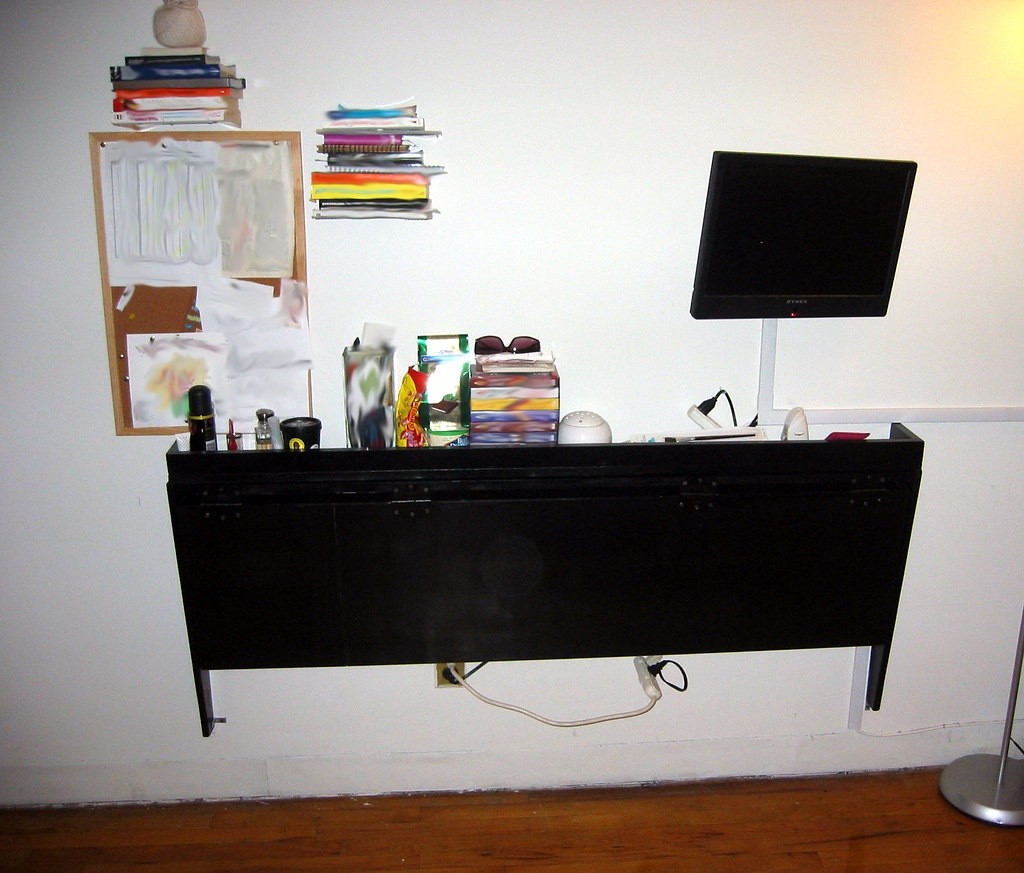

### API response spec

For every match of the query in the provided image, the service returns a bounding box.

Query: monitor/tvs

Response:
[688,151,918,317]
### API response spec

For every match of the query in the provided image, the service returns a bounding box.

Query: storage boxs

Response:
[418,334,471,446]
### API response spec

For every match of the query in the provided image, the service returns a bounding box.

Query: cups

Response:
[343,346,394,449]
[228,433,243,451]
[280,417,322,449]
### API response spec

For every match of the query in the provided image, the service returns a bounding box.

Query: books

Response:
[431,400,459,413]
[310,96,448,219]
[110,48,246,128]
[469,353,559,448]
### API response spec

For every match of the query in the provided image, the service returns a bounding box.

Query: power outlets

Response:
[436,663,465,688]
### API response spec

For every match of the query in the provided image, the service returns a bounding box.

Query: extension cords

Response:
[687,405,722,429]
[633,657,663,702]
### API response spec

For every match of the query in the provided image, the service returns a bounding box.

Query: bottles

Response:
[187,384,218,451]
[255,408,275,449]
